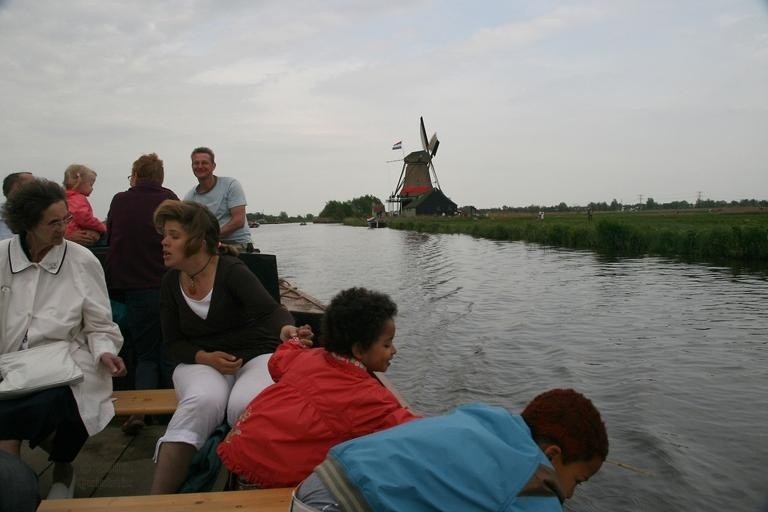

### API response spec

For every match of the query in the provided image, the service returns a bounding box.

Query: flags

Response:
[392,141,402,150]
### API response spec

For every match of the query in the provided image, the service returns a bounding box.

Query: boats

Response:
[368,221,385,228]
[249,223,260,228]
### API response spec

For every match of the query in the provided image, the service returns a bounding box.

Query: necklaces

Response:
[184,255,212,296]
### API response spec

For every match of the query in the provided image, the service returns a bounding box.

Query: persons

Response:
[0,177,128,501]
[150,200,314,495]
[0,172,97,248]
[107,154,180,433]
[289,388,609,512]
[182,147,255,256]
[61,164,108,247]
[216,287,424,491]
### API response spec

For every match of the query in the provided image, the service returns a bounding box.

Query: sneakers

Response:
[48,471,75,497]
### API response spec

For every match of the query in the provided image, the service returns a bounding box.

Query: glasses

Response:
[38,215,72,229]
